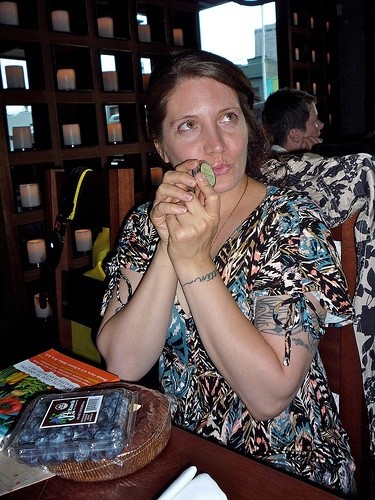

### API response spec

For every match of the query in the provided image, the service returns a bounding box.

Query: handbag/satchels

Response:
[39,166,94,309]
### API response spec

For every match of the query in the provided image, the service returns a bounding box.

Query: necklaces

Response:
[210,177,249,250]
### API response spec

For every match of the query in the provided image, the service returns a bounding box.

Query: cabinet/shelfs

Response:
[0,0,200,373]
[275,0,342,156]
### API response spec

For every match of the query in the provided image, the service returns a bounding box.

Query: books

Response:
[0,348,120,451]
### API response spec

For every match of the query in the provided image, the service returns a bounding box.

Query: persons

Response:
[260,88,325,157]
[95,50,357,496]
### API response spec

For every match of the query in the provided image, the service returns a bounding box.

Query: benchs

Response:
[46,152,374,499]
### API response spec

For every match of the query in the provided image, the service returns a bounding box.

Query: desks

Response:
[1,424,345,500]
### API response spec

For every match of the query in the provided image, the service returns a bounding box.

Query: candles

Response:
[294,12,333,126]
[34,293,50,318]
[0,2,184,47]
[12,122,123,149]
[5,66,151,91]
[27,239,47,264]
[75,229,93,252]
[19,183,41,207]
